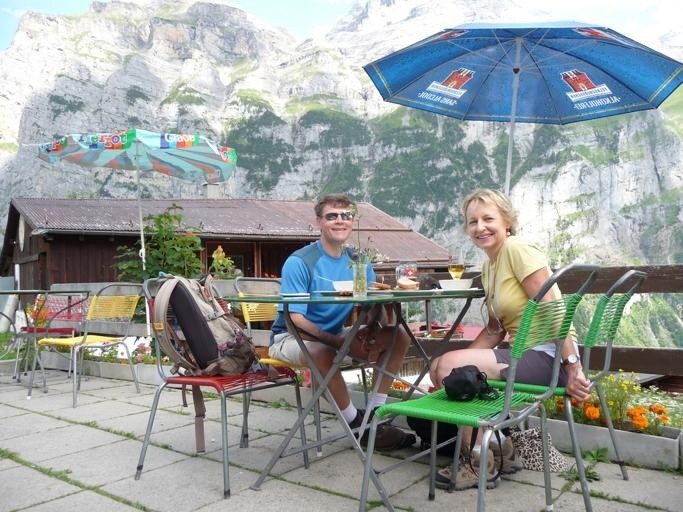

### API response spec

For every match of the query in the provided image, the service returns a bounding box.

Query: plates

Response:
[311,288,483,298]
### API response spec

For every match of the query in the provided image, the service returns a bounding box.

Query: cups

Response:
[447,249,465,280]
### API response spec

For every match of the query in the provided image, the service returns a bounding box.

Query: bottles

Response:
[393,262,419,291]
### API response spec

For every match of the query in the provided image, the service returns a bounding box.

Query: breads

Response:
[397,276,420,289]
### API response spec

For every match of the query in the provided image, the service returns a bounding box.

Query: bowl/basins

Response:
[333,281,353,291]
[438,279,473,288]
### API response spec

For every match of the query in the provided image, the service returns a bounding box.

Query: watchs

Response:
[562,354,580,367]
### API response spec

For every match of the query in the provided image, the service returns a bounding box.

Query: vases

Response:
[527,399,683,471]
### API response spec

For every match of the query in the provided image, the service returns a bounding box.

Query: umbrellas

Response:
[362,20,683,198]
[22,128,238,335]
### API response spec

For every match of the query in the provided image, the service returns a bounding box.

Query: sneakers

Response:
[435,446,501,491]
[349,407,416,451]
[489,436,523,474]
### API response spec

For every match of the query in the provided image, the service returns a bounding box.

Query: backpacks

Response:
[153,274,261,376]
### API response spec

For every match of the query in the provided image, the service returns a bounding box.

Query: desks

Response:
[0,289,90,397]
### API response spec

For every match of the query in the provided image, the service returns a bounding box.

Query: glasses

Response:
[481,294,503,336]
[317,212,355,220]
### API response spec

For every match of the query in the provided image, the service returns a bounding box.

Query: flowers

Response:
[528,369,683,436]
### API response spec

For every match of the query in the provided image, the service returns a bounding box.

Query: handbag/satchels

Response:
[444,365,488,400]
[510,428,577,473]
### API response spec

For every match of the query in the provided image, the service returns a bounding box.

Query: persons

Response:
[267,195,416,451]
[428,188,591,491]
